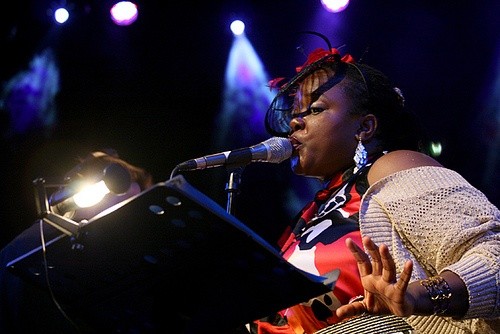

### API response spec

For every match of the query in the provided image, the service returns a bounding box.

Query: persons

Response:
[229,31,500,334]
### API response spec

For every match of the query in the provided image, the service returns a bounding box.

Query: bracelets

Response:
[421,276,452,315]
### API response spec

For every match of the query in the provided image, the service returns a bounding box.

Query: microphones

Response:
[36,175,81,187]
[177,137,293,172]
